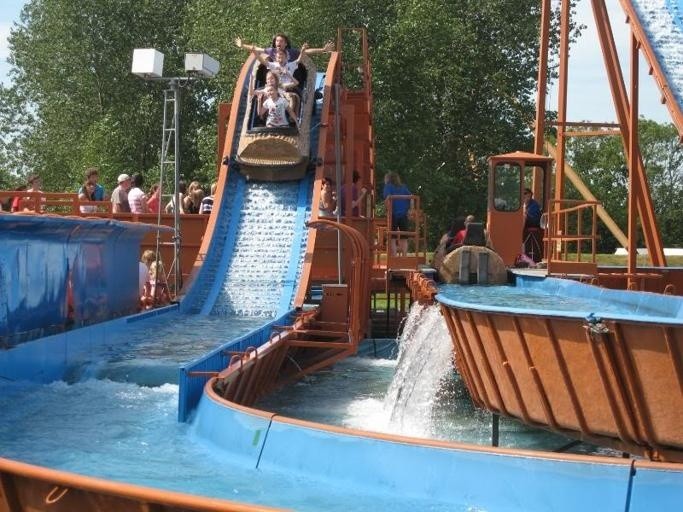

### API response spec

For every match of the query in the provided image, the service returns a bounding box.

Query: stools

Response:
[524,227,542,262]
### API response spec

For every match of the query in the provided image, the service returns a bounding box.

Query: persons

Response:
[234,33,336,129]
[23,176,47,212]
[144,249,169,310]
[139,261,151,299]
[522,188,542,227]
[382,170,414,257]
[447,215,476,254]
[10,184,27,213]
[318,177,336,216]
[341,171,367,217]
[78,167,217,215]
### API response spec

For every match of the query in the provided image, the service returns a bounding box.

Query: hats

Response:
[118,174,131,183]
